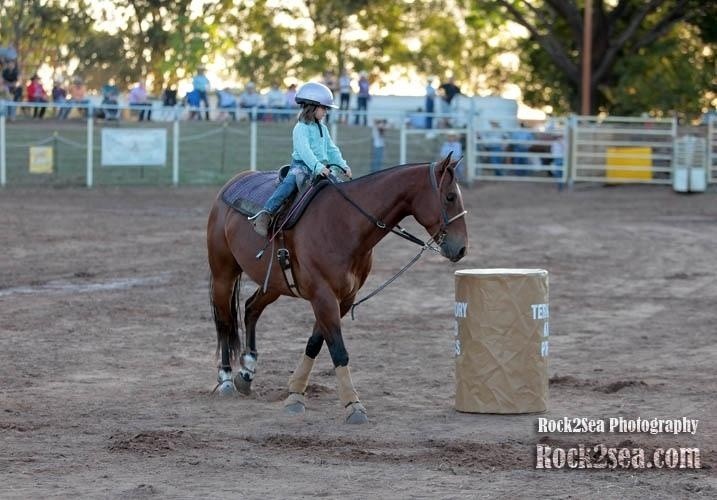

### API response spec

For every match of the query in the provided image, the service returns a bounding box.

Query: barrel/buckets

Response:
[456,267,551,415]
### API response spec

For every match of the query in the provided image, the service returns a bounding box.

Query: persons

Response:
[548,136,565,192]
[261,80,286,121]
[438,130,464,183]
[355,71,371,125]
[505,120,534,176]
[281,84,299,120]
[217,86,238,122]
[480,117,510,177]
[192,66,211,120]
[0,37,24,102]
[25,73,50,118]
[161,79,179,122]
[423,78,437,128]
[250,82,353,237]
[337,68,352,123]
[97,79,123,121]
[437,75,461,128]
[124,81,152,122]
[368,118,388,171]
[67,79,89,117]
[52,80,73,118]
[240,82,265,121]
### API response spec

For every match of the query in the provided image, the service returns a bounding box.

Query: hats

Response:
[30,74,84,82]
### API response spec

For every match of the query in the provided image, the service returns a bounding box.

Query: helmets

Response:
[294,81,339,109]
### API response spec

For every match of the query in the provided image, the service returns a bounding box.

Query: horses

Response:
[205,151,469,424]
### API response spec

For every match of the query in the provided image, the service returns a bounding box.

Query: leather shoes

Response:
[252,211,270,240]
